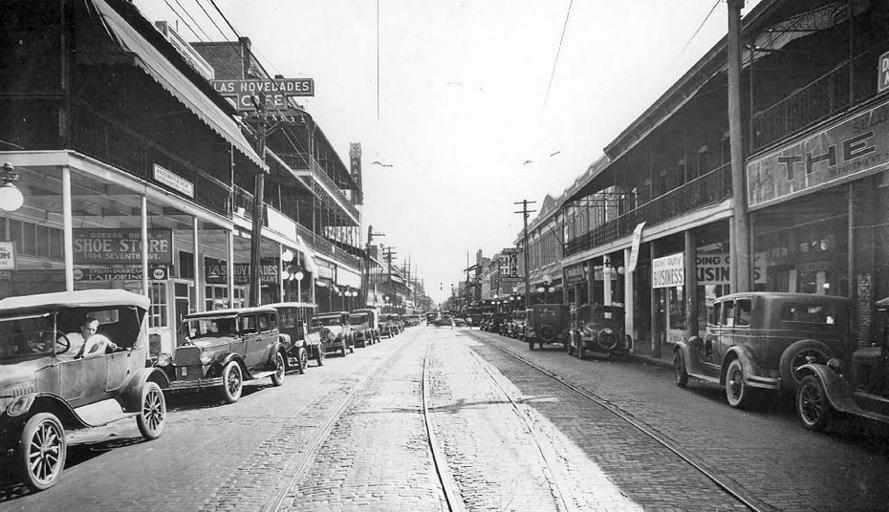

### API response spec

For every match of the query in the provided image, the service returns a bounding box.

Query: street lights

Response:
[281,250,307,301]
[338,284,359,309]
[537,274,555,304]
[488,293,502,307]
[508,286,521,307]
[384,294,392,308]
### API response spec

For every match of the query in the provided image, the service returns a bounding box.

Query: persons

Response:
[54,317,119,360]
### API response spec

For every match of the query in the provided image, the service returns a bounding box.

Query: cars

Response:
[669,289,852,416]
[0,286,172,492]
[317,310,355,358]
[567,304,634,364]
[350,308,436,350]
[437,307,529,346]
[261,302,326,374]
[793,294,888,437]
[149,305,286,403]
[526,302,574,353]
